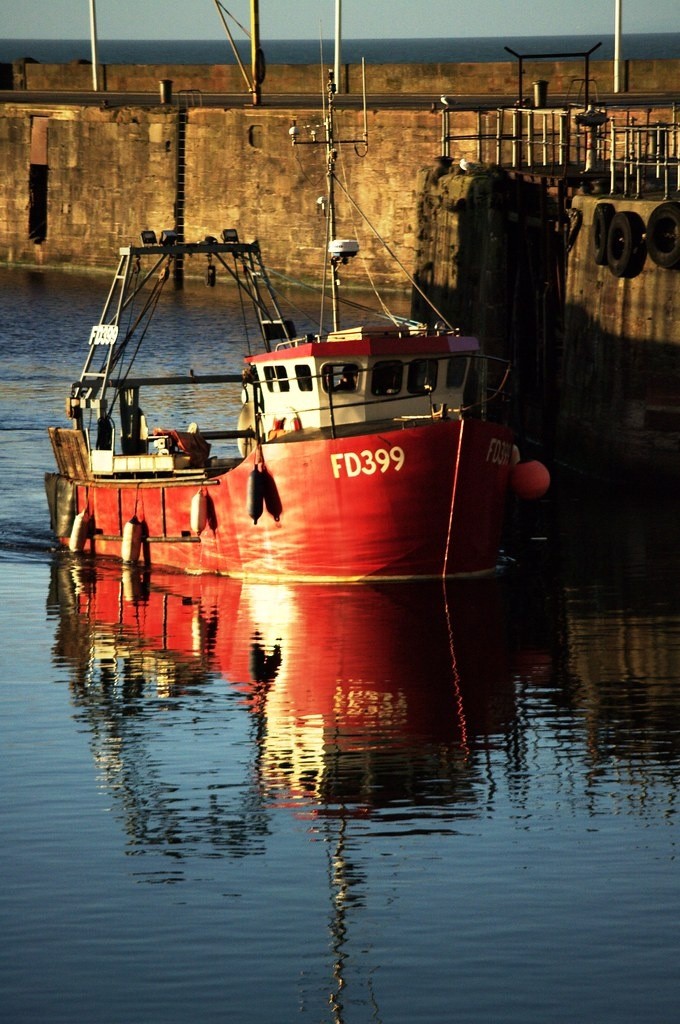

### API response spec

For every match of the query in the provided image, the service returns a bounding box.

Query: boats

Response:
[38,27,550,580]
[51,551,517,1024]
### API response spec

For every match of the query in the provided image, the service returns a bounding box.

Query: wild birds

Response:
[440,95,462,110]
[459,158,477,176]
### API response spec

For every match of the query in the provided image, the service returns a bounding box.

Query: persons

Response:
[336,365,357,390]
[328,68,334,81]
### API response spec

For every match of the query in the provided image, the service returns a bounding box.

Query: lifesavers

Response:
[591,199,680,279]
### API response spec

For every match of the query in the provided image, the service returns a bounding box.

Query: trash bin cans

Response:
[532,80,549,108]
[158,79,174,104]
